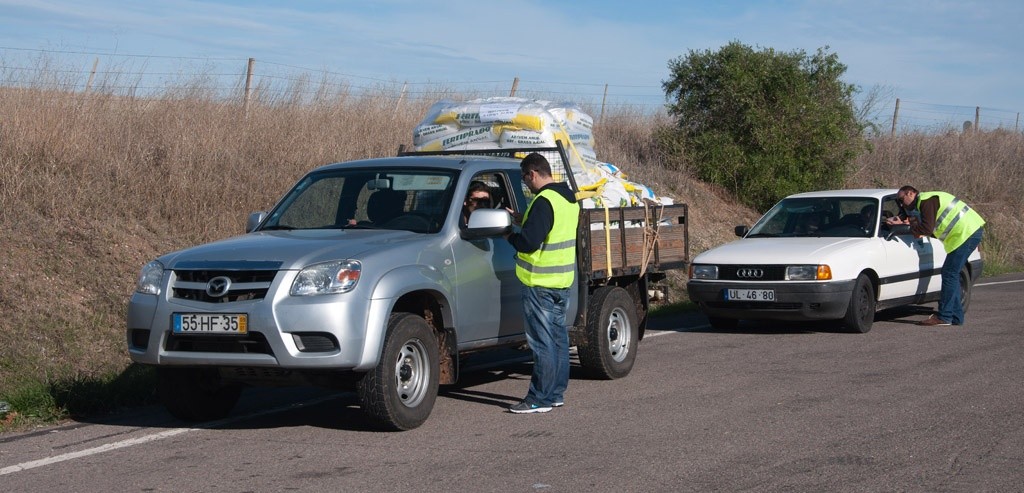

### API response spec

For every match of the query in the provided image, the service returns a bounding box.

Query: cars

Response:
[687,188,984,334]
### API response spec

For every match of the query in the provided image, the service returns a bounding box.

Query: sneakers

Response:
[551,401,564,407]
[510,399,553,414]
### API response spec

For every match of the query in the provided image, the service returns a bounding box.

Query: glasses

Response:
[522,169,539,181]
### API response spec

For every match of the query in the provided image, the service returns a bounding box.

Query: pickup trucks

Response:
[126,140,690,434]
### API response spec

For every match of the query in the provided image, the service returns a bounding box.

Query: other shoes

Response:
[919,314,951,326]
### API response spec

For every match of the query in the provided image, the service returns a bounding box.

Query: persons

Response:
[860,205,888,233]
[793,212,821,235]
[458,180,492,231]
[345,189,408,228]
[885,185,986,326]
[504,152,581,414]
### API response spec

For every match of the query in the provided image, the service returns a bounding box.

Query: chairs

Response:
[355,189,405,228]
